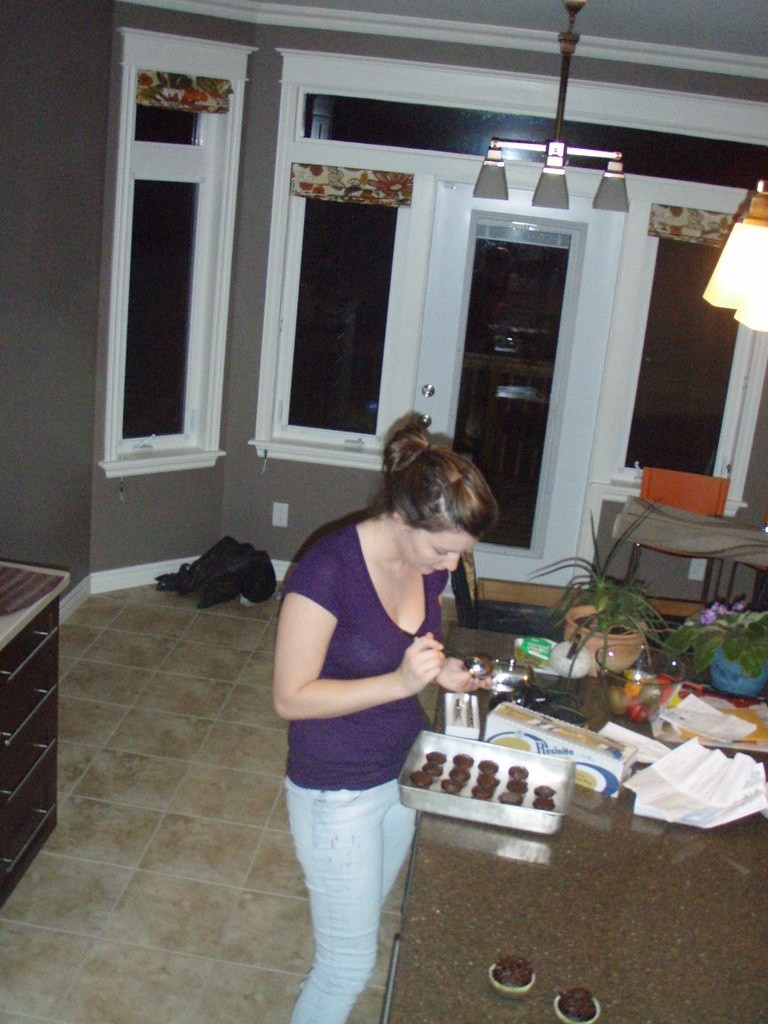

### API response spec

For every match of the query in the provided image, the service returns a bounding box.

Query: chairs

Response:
[621,466,731,600]
[453,548,561,642]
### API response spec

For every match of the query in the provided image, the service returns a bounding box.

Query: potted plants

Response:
[523,504,690,695]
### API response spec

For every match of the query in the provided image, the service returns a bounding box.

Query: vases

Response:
[709,645,768,700]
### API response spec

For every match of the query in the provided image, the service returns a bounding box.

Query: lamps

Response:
[703,179,768,333]
[472,0,629,213]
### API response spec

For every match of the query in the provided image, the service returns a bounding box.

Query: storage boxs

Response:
[484,700,639,799]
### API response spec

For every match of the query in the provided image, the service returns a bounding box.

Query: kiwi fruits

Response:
[639,679,662,704]
[607,685,627,715]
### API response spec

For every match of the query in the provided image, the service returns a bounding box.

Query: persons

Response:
[269,417,498,1024]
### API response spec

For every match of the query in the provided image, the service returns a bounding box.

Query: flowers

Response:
[659,587,768,679]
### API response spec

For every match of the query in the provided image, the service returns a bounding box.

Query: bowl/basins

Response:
[593,646,687,727]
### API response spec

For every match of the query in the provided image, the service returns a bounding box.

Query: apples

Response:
[628,700,649,721]
[657,675,672,701]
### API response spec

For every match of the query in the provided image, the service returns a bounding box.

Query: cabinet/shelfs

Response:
[0,592,63,910]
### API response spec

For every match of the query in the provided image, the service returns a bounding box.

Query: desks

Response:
[612,495,768,612]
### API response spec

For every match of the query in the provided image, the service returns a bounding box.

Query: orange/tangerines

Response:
[625,676,647,698]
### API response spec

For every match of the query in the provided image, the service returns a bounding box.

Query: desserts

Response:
[558,987,597,1021]
[410,751,556,810]
[492,957,533,987]
